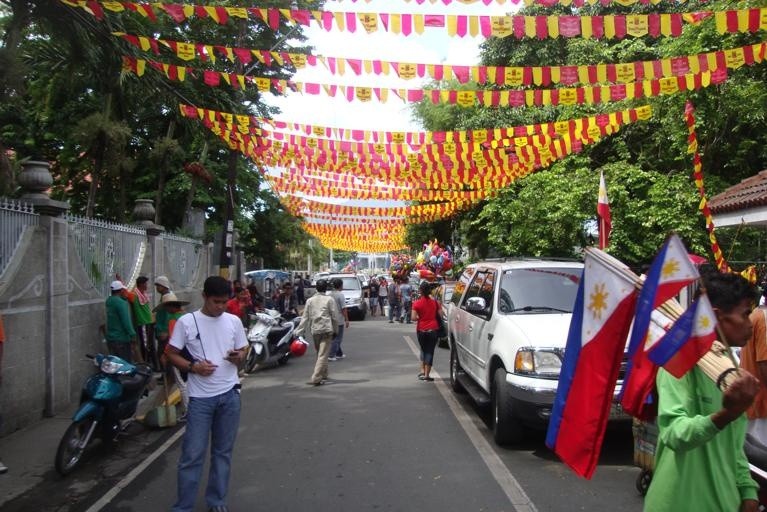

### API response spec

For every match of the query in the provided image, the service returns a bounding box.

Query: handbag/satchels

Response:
[176,310,206,383]
[436,318,448,338]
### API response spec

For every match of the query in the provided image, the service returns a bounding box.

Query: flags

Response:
[545,168,719,481]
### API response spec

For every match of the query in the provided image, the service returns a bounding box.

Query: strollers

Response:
[278,307,305,339]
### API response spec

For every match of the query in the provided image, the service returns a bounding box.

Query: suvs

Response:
[327,272,371,322]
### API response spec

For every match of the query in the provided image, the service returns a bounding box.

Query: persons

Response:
[368,274,446,381]
[104,274,194,424]
[739,284,767,473]
[642,272,761,512]
[224,273,349,387]
[164,277,250,512]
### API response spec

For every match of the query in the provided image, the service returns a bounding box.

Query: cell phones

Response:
[229,351,238,357]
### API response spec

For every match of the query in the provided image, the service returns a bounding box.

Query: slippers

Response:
[305,376,328,385]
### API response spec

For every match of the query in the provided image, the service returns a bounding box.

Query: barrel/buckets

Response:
[383,305,389,317]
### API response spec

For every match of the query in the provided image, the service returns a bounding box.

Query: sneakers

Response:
[328,354,347,361]
[370,313,412,324]
[418,373,434,382]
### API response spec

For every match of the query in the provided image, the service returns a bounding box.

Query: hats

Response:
[110,280,128,292]
[151,292,192,314]
[154,276,171,290]
[283,282,294,290]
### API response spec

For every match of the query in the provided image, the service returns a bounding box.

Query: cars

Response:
[432,279,458,347]
[308,271,445,289]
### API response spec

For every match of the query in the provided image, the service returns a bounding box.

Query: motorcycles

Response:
[260,305,285,326]
[240,311,307,374]
[51,350,163,479]
[272,283,285,305]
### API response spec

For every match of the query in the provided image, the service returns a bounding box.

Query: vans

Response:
[446,253,647,451]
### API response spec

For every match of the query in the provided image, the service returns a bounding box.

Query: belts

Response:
[232,383,243,390]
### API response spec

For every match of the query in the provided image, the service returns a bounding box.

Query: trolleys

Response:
[633,416,662,495]
